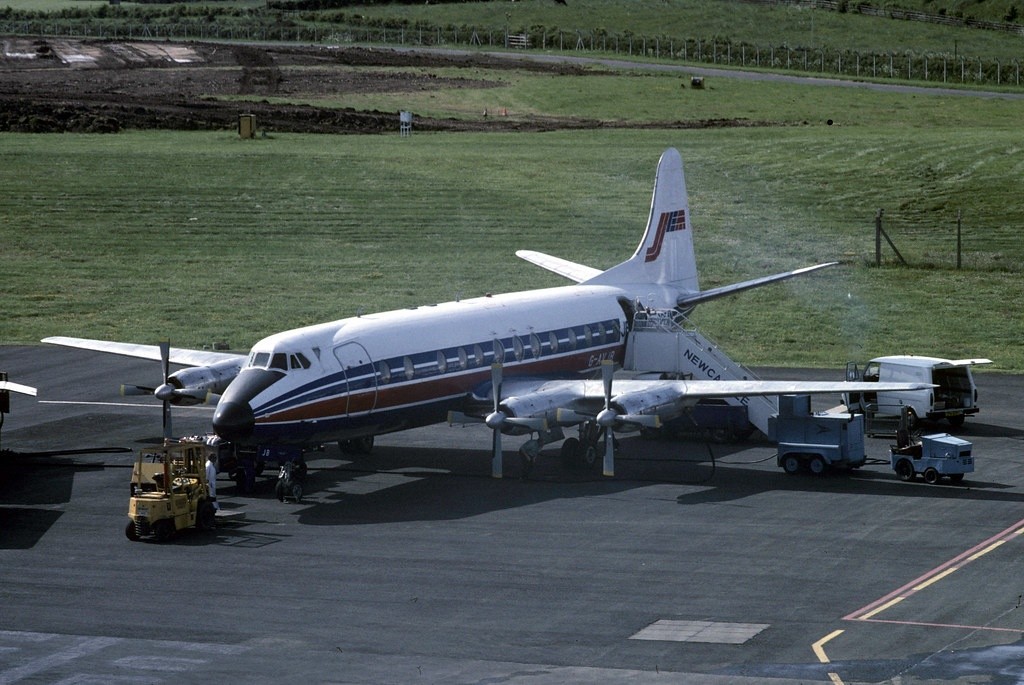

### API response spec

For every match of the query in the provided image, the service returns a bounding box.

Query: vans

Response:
[840,353,993,430]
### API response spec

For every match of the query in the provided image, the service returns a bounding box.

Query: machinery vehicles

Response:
[125,438,216,543]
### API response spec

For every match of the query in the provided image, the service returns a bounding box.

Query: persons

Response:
[205,451,218,510]
[519,438,544,480]
[484,108,488,121]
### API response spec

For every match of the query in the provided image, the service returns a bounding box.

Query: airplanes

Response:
[37,148,940,502]
